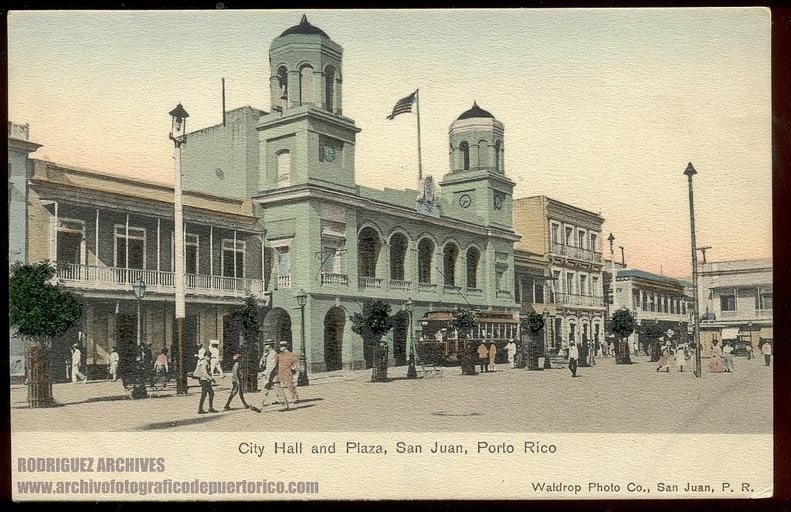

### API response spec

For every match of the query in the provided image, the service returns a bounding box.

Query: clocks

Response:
[458,194,471,208]
[494,194,502,210]
[319,140,337,162]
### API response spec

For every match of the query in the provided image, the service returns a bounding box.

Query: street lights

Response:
[587,309,596,365]
[404,297,418,379]
[131,275,148,347]
[748,322,754,357]
[167,101,190,397]
[294,287,311,385]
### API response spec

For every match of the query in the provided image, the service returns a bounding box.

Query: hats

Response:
[278,341,289,346]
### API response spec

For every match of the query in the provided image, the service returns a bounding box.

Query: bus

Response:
[416,309,518,366]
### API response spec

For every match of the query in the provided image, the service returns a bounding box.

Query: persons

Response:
[67,338,772,414]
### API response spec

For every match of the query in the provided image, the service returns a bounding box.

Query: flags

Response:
[386,93,414,120]
